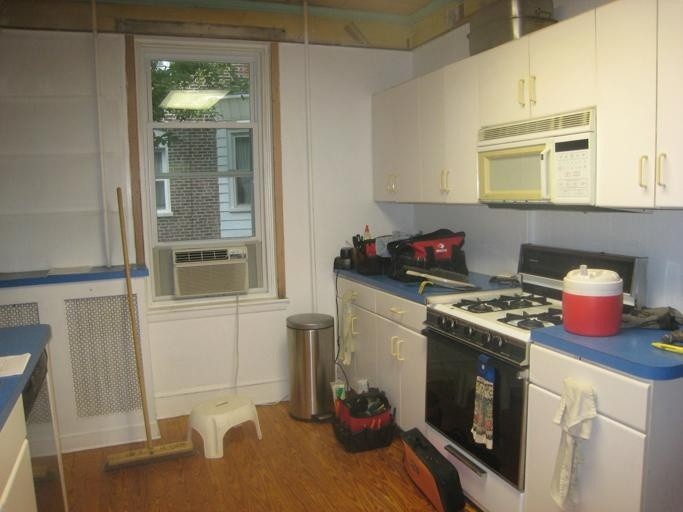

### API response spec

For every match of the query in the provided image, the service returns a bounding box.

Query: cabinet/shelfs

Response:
[336,277,375,388]
[371,76,422,205]
[477,8,595,123]
[523,345,683,512]
[422,423,523,512]
[373,288,426,436]
[422,59,478,204]
[595,1,682,211]
[0,83,111,274]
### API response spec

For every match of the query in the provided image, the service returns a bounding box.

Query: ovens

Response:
[423,307,532,493]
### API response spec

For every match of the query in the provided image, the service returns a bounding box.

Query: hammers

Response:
[663,328,683,346]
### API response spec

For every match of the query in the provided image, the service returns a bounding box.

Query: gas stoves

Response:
[427,289,563,342]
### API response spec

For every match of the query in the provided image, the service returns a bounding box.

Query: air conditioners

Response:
[169,244,250,299]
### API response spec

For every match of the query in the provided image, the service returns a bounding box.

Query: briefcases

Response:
[402,428,465,511]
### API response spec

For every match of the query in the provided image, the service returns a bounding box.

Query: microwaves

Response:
[477,129,599,210]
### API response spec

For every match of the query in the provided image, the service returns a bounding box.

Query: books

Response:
[0,352,31,378]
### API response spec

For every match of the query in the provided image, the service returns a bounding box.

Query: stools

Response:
[187,393,263,460]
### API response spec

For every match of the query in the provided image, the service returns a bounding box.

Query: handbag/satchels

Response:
[352,233,413,274]
[387,228,468,282]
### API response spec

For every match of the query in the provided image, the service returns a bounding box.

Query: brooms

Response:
[102,186,194,472]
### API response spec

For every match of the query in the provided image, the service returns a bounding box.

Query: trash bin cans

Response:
[286,314,335,422]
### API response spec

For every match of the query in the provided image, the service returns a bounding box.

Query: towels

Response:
[336,290,356,367]
[550,377,598,512]
[469,354,494,451]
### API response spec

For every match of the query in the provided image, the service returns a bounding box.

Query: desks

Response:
[0,261,149,512]
